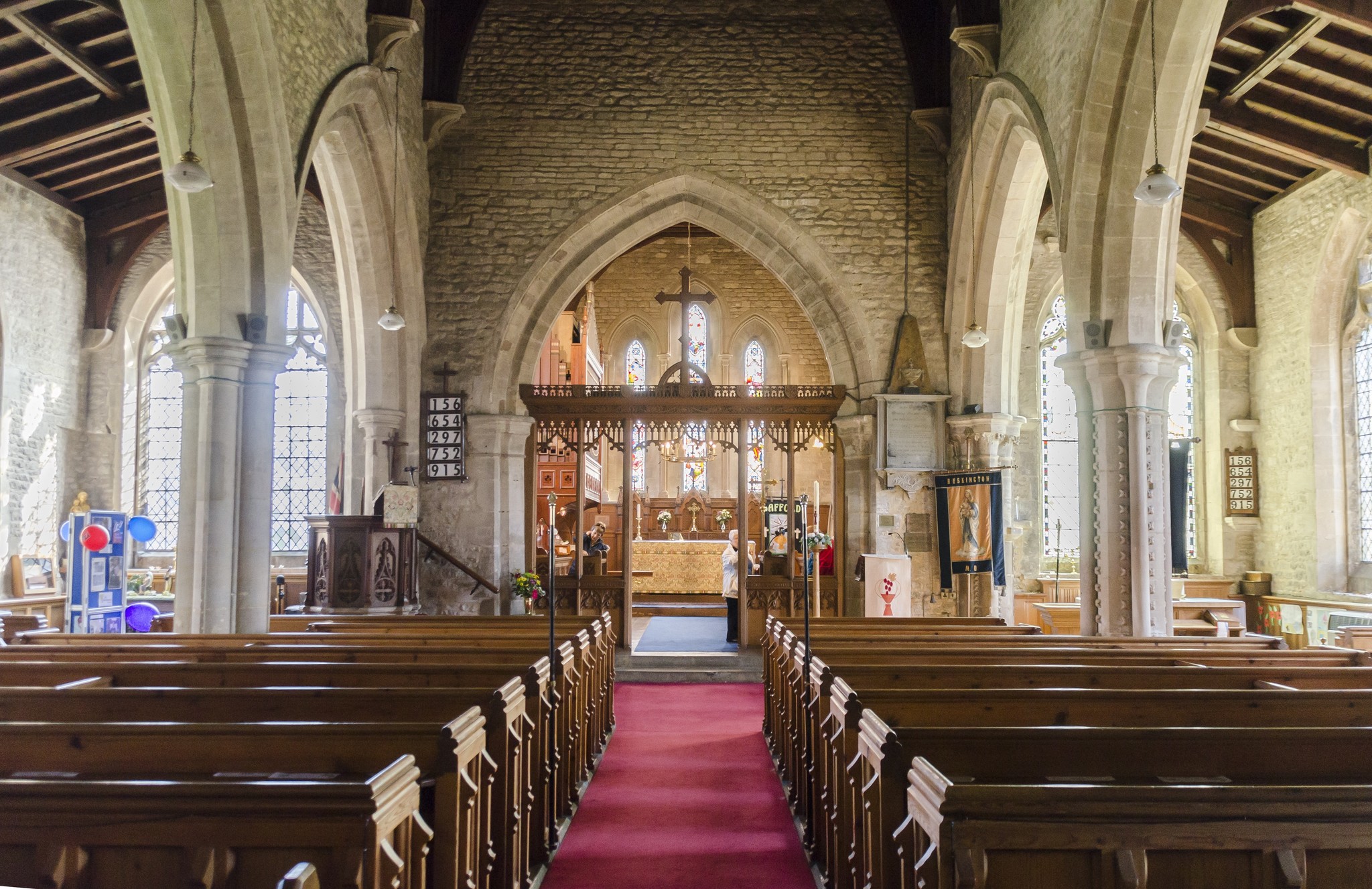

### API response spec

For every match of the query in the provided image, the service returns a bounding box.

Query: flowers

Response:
[657,509,672,523]
[715,509,732,525]
[511,568,546,614]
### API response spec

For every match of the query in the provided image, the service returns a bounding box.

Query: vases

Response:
[721,521,725,532]
[662,521,667,532]
[524,597,534,615]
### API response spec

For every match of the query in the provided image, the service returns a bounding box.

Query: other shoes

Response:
[727,638,738,642]
[566,600,572,609]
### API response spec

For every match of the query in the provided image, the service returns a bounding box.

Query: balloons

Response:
[125,602,160,632]
[128,516,157,542]
[60,521,69,542]
[80,524,111,552]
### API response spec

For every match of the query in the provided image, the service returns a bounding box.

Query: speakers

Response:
[1083,320,1105,349]
[246,314,268,344]
[1164,320,1187,347]
[163,313,187,343]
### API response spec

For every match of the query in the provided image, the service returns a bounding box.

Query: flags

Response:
[328,453,344,515]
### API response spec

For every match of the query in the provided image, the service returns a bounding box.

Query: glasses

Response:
[593,524,605,532]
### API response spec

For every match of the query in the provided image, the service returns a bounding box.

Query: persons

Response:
[164,566,173,592]
[138,572,153,595]
[806,526,834,576]
[70,491,90,513]
[568,521,610,575]
[722,529,754,642]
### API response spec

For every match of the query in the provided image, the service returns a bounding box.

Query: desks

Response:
[1035,598,1245,639]
[630,539,756,594]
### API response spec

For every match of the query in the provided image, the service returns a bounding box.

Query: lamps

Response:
[662,440,717,462]
[164,0,215,193]
[379,65,404,331]
[962,75,993,348]
[1134,0,1182,205]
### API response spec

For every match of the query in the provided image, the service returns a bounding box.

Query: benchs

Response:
[760,616,1372,889]
[0,612,617,889]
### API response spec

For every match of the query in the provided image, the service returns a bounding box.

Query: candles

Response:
[637,504,641,518]
[814,481,819,533]
